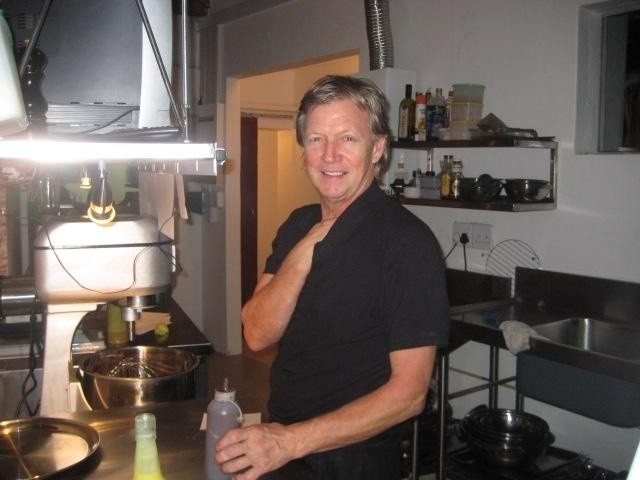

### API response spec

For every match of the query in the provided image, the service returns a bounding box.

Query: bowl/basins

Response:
[455,407,559,470]
[502,178,554,206]
[456,173,504,203]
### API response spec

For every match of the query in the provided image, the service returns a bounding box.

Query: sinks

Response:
[516,314,638,429]
[449,300,569,352]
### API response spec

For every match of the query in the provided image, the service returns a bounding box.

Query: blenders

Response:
[31,211,202,420]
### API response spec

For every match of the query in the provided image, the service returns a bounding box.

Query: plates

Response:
[2,413,101,480]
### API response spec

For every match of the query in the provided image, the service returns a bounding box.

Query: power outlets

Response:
[452,220,473,248]
[473,222,494,250]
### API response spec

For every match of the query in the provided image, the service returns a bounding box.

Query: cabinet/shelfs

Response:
[382,135,560,214]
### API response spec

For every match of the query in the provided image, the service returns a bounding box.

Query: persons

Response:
[213,73,452,479]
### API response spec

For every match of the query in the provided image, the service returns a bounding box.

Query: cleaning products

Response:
[414,94,427,140]
[133,412,160,479]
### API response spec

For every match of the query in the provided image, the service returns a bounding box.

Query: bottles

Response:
[129,415,166,480]
[390,152,462,199]
[396,82,453,141]
